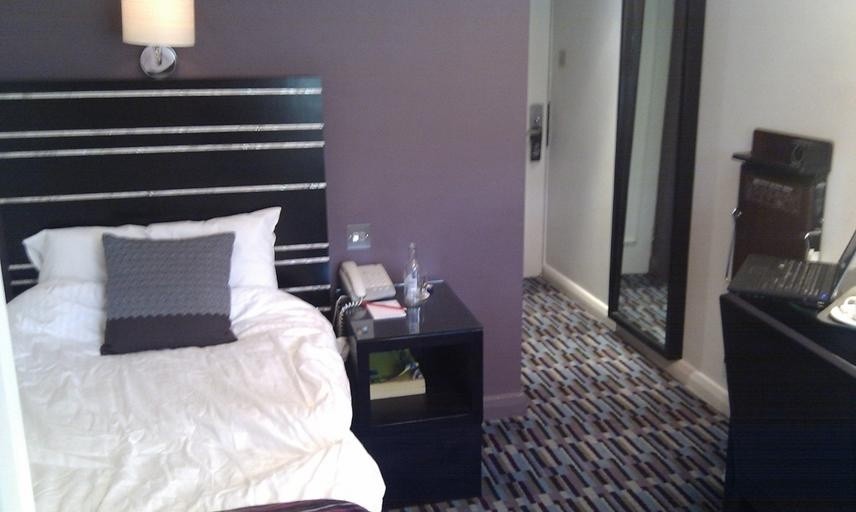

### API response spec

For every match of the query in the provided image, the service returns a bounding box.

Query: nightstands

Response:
[335,279,483,512]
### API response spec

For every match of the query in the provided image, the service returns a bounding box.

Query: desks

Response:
[719,292,854,509]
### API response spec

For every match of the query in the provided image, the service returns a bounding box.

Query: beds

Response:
[0,76,370,510]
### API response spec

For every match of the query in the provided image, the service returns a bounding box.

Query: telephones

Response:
[338,260,397,304]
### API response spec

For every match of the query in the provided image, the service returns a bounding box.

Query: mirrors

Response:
[607,1,706,362]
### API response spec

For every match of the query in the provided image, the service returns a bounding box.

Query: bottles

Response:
[402,239,423,309]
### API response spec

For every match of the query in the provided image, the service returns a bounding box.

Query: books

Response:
[366,298,407,321]
[368,348,428,401]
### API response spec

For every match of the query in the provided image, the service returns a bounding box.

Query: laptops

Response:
[728,231,856,308]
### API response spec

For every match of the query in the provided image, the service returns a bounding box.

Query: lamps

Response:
[120,1,195,81]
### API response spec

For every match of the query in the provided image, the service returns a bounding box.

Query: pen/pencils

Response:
[362,302,408,311]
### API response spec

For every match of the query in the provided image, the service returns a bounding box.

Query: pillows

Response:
[22,206,284,354]
